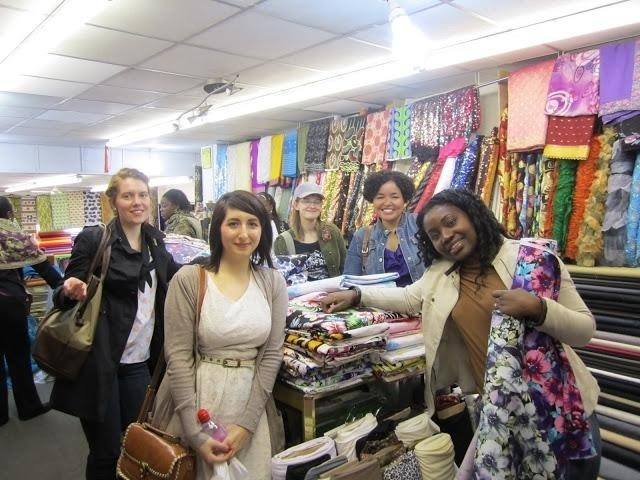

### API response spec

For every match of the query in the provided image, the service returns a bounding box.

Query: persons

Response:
[273,180,346,284]
[157,188,203,243]
[340,169,432,292]
[257,193,289,242]
[1,196,65,426]
[49,167,184,480]
[149,189,288,480]
[319,188,602,480]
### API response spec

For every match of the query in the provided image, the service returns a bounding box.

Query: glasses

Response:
[298,198,325,204]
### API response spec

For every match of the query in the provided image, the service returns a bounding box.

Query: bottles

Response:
[197,408,228,442]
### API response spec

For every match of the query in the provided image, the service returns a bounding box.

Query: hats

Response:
[291,183,324,199]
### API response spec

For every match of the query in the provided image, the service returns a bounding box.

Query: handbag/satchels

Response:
[31,222,112,379]
[114,264,206,480]
